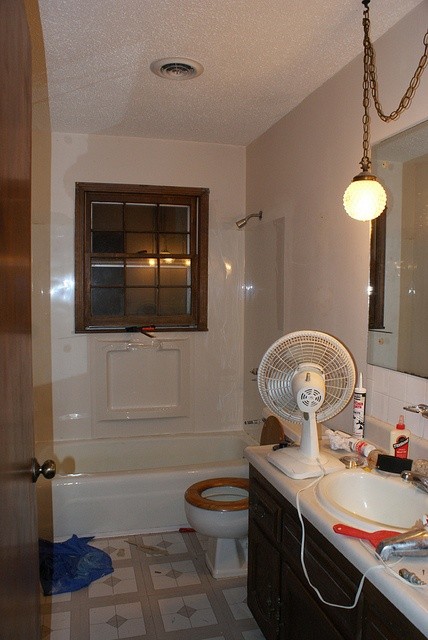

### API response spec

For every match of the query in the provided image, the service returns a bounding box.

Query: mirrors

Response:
[367,118,428,380]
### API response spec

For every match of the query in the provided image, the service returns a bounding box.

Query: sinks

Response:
[327,470,427,531]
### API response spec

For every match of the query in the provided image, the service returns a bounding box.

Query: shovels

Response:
[333,522,403,550]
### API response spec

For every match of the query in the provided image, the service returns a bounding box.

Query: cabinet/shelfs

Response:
[248,437,365,640]
[365,579,428,640]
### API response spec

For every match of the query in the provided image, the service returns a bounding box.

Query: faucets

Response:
[402,457,427,481]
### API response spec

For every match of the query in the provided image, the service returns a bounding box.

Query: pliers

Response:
[126,325,155,332]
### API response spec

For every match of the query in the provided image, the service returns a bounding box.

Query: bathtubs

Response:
[33,428,264,542]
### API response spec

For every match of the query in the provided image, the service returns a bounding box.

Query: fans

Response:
[258,330,356,480]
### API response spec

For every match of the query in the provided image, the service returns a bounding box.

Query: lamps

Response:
[236,211,262,229]
[342,1,428,221]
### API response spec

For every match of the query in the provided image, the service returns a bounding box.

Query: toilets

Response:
[184,476,253,577]
